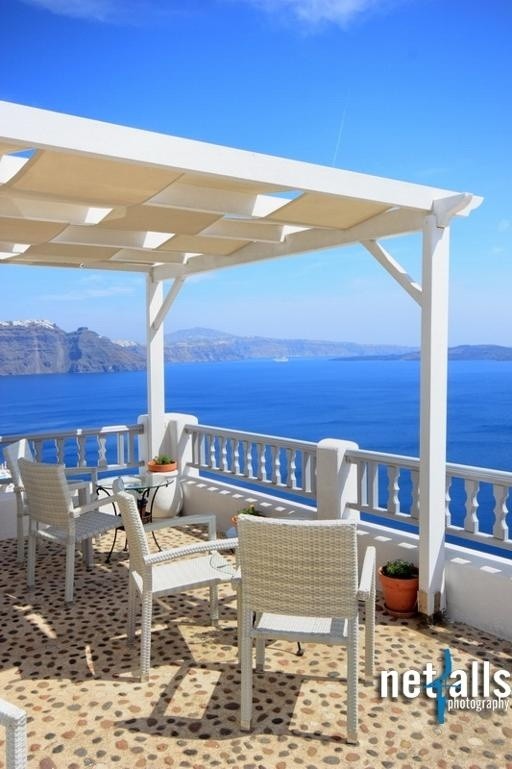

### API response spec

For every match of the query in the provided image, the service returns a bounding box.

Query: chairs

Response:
[235,515,375,743]
[116,491,243,682]
[4,439,99,566]
[18,459,125,602]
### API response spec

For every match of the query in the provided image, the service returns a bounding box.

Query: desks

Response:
[95,475,174,564]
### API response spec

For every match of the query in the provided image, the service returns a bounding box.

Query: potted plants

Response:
[232,507,265,532]
[379,561,419,612]
[148,457,176,471]
